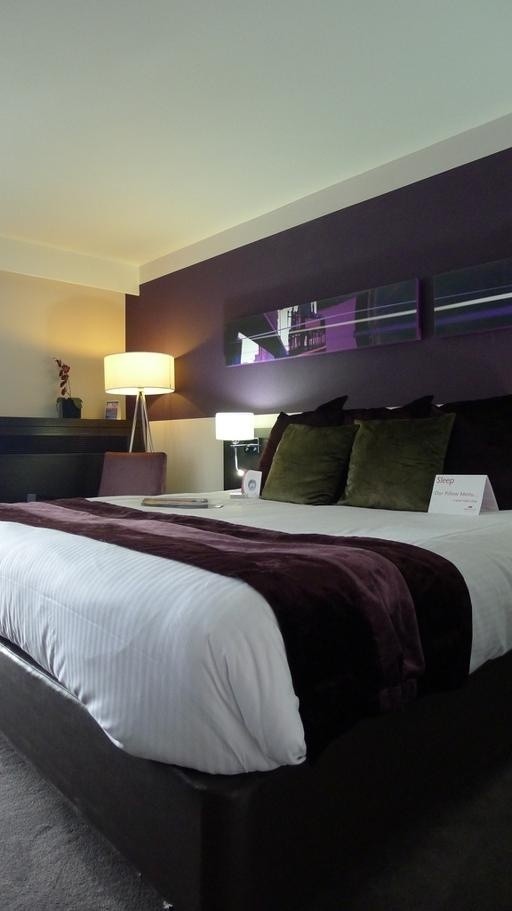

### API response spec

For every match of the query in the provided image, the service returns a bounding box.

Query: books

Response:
[142,496,209,509]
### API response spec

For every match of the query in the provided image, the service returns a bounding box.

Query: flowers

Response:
[56,359,83,409]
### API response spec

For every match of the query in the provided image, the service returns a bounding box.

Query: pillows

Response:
[256,393,512,512]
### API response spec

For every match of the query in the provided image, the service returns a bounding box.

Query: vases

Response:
[62,398,81,418]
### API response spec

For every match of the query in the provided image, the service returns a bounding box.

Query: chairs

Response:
[97,452,167,496]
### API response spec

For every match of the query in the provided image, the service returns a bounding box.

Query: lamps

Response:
[103,351,175,452]
[214,411,261,476]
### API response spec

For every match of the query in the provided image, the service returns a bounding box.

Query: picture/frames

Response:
[104,400,120,421]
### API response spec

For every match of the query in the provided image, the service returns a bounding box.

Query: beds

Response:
[0,489,512,911]
